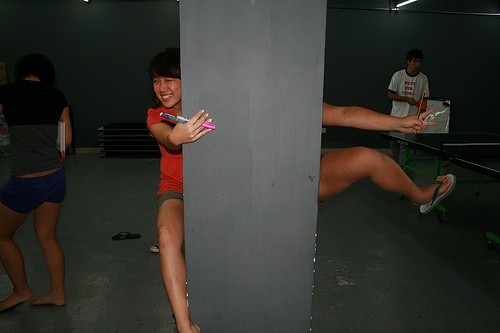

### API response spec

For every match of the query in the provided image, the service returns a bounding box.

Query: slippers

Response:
[111,231,142,241]
[419,174,457,215]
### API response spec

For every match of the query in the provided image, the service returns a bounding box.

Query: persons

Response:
[387,48,430,161]
[0,52,75,313]
[319,101,458,216]
[144,47,217,333]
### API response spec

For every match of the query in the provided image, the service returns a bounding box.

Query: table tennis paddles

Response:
[416,99,427,111]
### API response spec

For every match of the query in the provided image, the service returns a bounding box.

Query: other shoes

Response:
[150,244,160,253]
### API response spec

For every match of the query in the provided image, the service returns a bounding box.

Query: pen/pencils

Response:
[417,91,426,119]
[159,112,217,130]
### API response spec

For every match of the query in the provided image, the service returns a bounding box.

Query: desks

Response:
[384,132,500,244]
